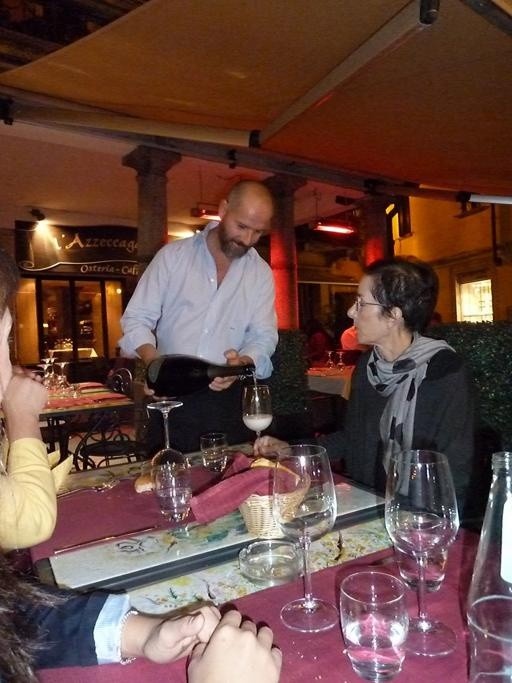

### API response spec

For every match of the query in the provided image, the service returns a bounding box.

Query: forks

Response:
[56,476,120,502]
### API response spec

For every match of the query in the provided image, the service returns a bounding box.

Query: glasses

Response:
[354,294,392,312]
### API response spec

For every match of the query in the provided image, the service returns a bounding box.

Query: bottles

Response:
[467,449,511,624]
[147,353,256,398]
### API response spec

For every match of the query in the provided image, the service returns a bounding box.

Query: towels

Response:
[187,453,296,524]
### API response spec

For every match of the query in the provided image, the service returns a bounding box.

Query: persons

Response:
[297,316,333,370]
[1,234,60,556]
[252,249,484,524]
[338,324,375,353]
[1,545,287,683]
[116,176,282,459]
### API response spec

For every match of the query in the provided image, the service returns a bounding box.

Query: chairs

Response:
[64,367,133,472]
[80,398,161,471]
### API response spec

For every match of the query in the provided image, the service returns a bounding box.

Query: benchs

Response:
[424,320,512,534]
[257,328,311,435]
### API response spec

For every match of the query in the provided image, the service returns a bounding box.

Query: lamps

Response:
[311,189,359,237]
[0,207,45,236]
[383,194,412,241]
[190,161,223,222]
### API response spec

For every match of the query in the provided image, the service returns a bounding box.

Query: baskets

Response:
[238,458,312,539]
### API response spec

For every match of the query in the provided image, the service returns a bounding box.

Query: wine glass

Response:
[273,444,339,632]
[146,399,194,492]
[385,449,459,659]
[242,384,274,459]
[336,351,346,371]
[55,360,71,392]
[325,350,335,368]
[38,363,54,390]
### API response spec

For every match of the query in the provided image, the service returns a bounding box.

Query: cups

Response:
[200,433,228,472]
[394,546,450,592]
[154,464,192,522]
[341,570,409,683]
[468,596,511,683]
